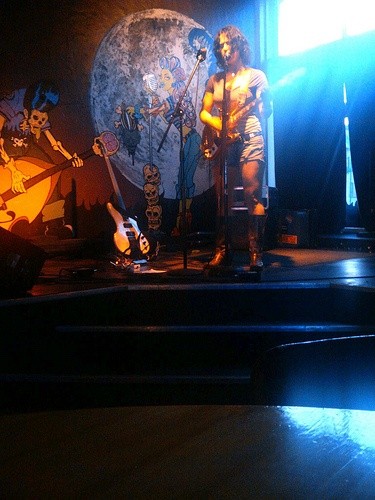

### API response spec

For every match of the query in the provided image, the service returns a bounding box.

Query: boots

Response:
[207,216,232,267]
[247,214,267,271]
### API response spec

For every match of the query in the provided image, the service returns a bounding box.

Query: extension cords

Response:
[116,258,140,273]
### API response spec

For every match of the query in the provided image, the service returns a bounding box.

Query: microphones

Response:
[197,48,207,62]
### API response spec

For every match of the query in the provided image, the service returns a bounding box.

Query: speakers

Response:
[0,226,50,293]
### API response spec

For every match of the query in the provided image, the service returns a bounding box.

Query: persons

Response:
[200,26,272,275]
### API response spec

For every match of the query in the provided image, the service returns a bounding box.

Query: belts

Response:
[241,131,263,139]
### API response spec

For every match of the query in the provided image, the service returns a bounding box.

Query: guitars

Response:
[202,68,306,160]
[95,139,149,255]
